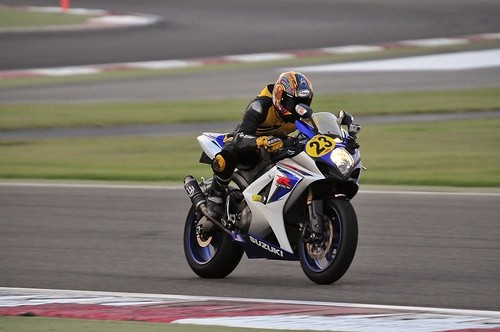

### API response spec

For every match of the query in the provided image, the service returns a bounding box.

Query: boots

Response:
[205,172,233,219]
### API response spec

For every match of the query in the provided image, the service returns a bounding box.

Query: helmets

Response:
[272,71,314,116]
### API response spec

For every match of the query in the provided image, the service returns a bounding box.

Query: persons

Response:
[206,71,314,217]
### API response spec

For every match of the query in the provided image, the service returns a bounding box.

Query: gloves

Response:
[255,135,283,153]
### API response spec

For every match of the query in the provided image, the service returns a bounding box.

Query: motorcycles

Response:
[183,110,366,284]
